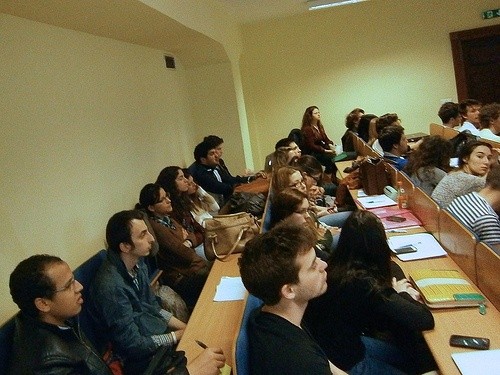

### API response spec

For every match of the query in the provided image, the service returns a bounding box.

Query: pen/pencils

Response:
[194,339,207,349]
[401,242,421,247]
[366,201,384,203]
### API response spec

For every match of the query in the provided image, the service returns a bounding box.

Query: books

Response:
[408,269,487,309]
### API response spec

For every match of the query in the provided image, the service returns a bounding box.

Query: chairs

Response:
[0,122,500,375]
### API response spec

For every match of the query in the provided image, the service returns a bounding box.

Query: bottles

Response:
[396,182,408,209]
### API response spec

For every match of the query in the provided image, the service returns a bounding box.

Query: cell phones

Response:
[392,246,417,253]
[386,216,406,222]
[449,335,491,350]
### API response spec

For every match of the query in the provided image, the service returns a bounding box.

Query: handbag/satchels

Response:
[358,155,389,195]
[201,211,264,260]
[227,189,267,219]
[235,175,269,196]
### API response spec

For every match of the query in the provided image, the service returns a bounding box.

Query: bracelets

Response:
[171,332,177,344]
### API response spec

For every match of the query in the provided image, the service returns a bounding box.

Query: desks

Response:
[175,253,249,375]
[334,158,500,375]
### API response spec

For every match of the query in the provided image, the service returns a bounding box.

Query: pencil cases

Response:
[383,185,398,202]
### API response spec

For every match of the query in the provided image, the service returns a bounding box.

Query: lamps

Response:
[306,0,363,10]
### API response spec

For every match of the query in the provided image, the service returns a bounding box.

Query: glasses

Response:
[52,279,77,294]
[287,178,307,188]
[153,192,170,205]
[292,208,313,217]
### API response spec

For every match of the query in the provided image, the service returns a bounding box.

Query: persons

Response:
[134,99,500,325]
[80,210,188,359]
[9,254,226,375]
[300,210,434,375]
[237,222,442,375]
